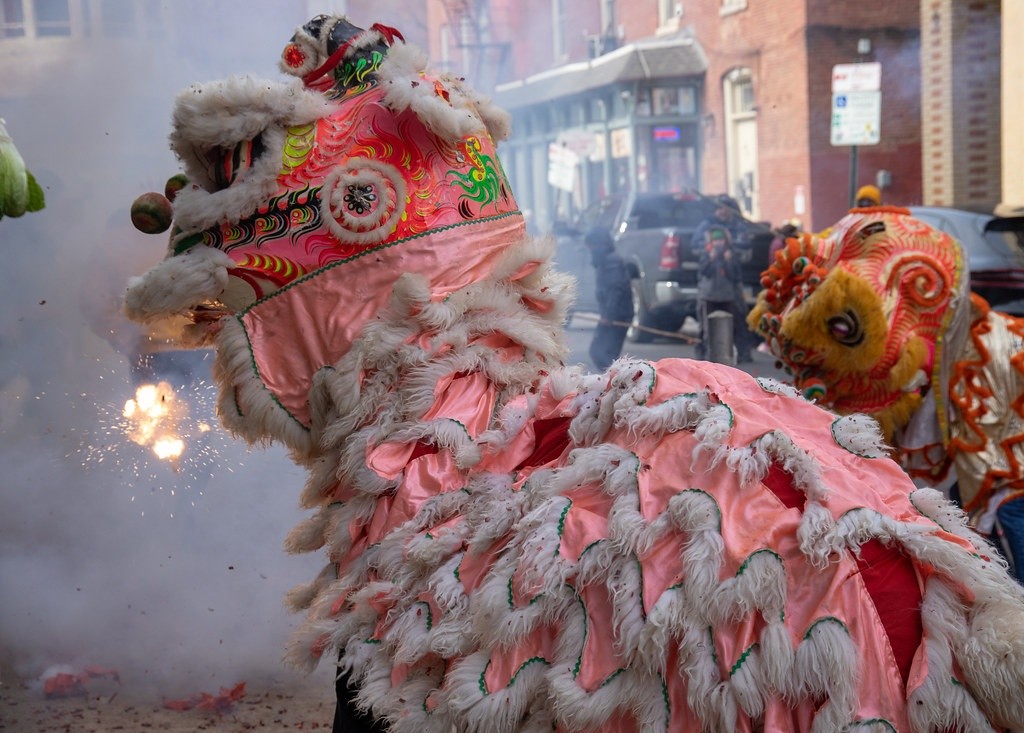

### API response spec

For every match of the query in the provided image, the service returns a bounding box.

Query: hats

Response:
[585,226,615,247]
[711,229,726,240]
[713,195,735,208]
[856,185,881,206]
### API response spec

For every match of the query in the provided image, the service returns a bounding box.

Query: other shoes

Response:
[737,356,753,363]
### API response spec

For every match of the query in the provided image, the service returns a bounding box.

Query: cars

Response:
[905,204,1024,307]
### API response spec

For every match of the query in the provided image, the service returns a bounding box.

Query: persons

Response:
[124,11,1024,733]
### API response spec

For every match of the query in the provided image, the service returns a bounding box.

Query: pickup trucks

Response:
[563,195,775,338]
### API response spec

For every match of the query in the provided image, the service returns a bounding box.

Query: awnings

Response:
[489,29,706,109]
